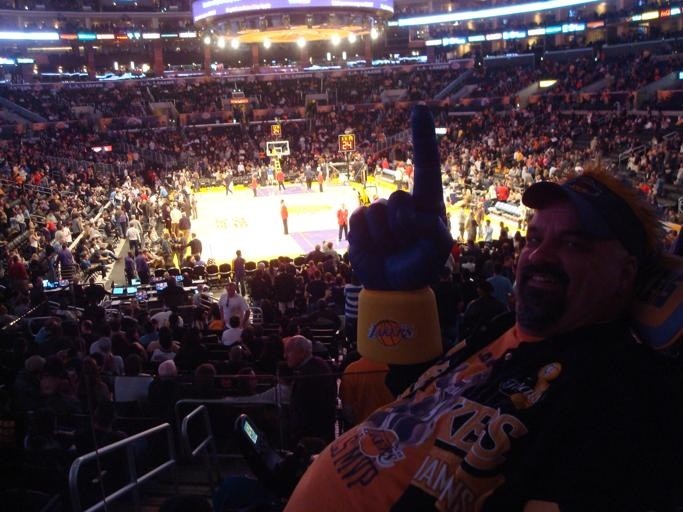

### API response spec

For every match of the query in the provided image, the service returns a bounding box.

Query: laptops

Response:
[42,279,58,289]
[112,275,199,307]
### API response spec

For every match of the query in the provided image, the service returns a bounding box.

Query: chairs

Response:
[0,106,508,512]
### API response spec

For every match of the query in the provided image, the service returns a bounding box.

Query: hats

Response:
[522,175,650,274]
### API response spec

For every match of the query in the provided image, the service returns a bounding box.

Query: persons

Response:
[1,0,683,512]
